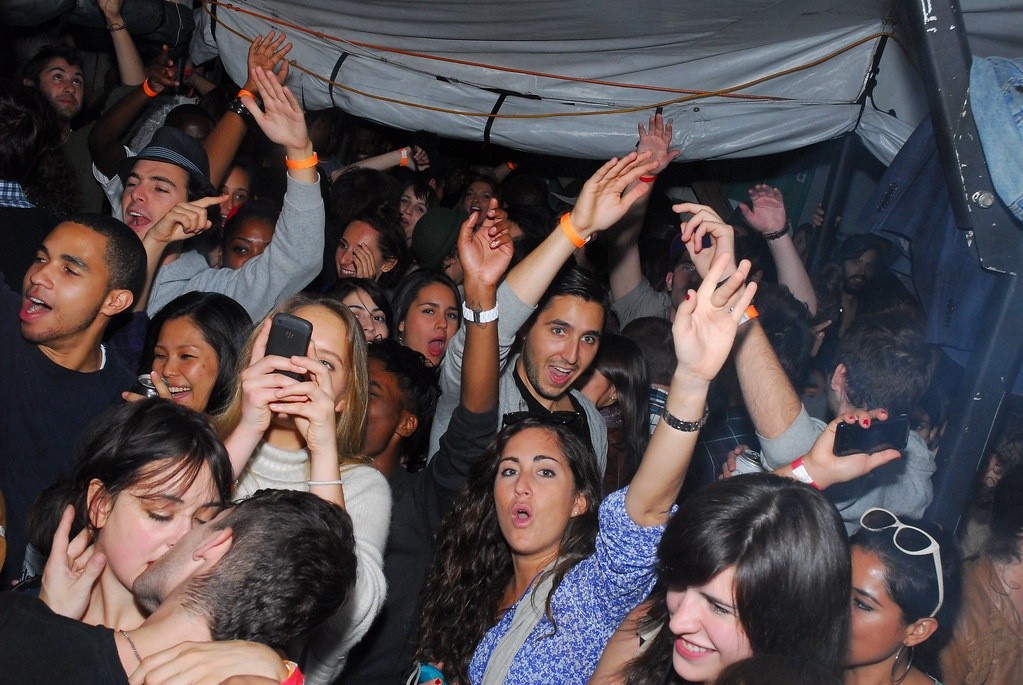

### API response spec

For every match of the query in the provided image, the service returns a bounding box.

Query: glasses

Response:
[501,411,583,434]
[859,507,943,619]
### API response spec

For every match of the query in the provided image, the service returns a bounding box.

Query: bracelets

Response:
[791,457,822,491]
[400,148,408,167]
[713,278,742,293]
[662,402,710,433]
[227,90,262,120]
[507,161,514,170]
[735,305,758,325]
[308,480,343,486]
[143,78,157,97]
[763,224,791,240]
[560,214,590,248]
[108,22,128,32]
[640,175,656,184]
[286,152,318,170]
[462,302,499,324]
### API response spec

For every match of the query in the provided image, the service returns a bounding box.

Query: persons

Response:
[0,0,1023,685]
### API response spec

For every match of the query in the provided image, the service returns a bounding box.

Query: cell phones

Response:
[833,415,911,458]
[264,312,313,380]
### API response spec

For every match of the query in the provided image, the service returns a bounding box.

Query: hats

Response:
[117,125,218,197]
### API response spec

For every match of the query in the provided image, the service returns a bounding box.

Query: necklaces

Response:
[120,629,143,665]
